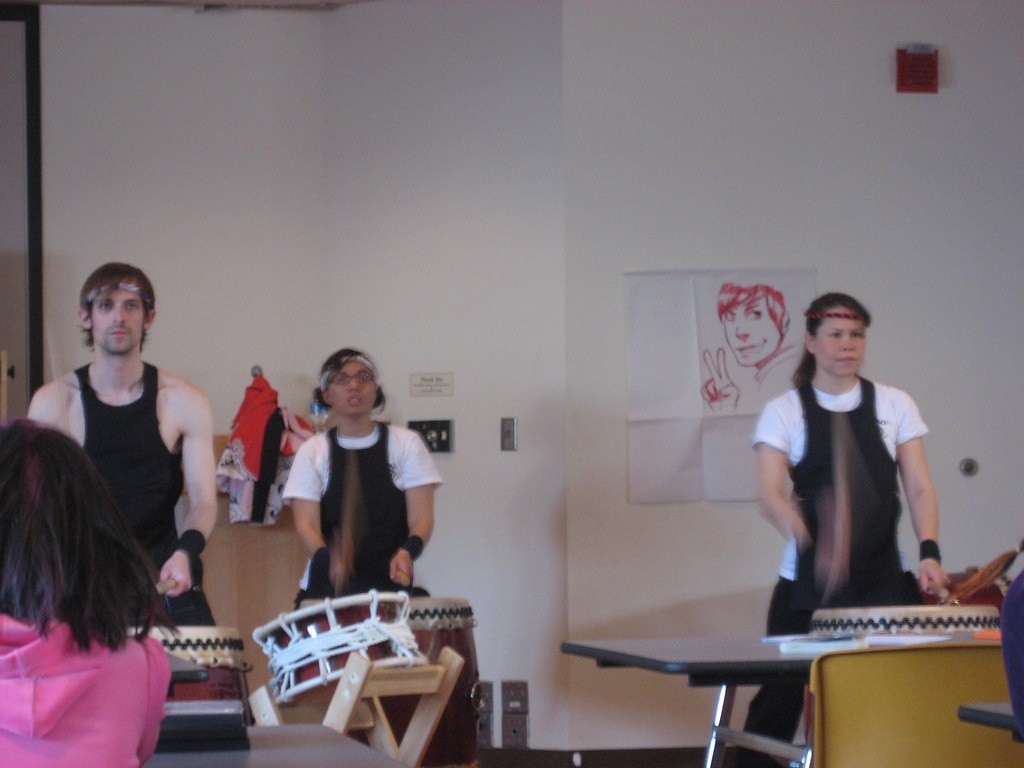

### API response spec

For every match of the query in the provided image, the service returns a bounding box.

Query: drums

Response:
[124,624,252,726]
[803,603,1000,745]
[250,586,429,709]
[295,593,480,768]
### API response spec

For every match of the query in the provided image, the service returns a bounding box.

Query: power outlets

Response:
[476,678,529,749]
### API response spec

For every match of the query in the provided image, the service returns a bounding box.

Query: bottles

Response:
[310,388,328,435]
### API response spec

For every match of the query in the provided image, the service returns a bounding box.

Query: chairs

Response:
[788,572,1024,768]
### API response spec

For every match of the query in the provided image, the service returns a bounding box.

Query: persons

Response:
[0,420,178,768]
[739,293,950,768]
[26,263,215,627]
[281,349,443,615]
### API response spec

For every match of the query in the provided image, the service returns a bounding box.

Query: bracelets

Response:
[176,529,206,557]
[402,536,424,562]
[920,540,940,563]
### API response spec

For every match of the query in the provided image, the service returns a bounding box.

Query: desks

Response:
[554,631,1000,767]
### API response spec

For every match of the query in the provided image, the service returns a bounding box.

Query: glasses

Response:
[324,369,372,387]
